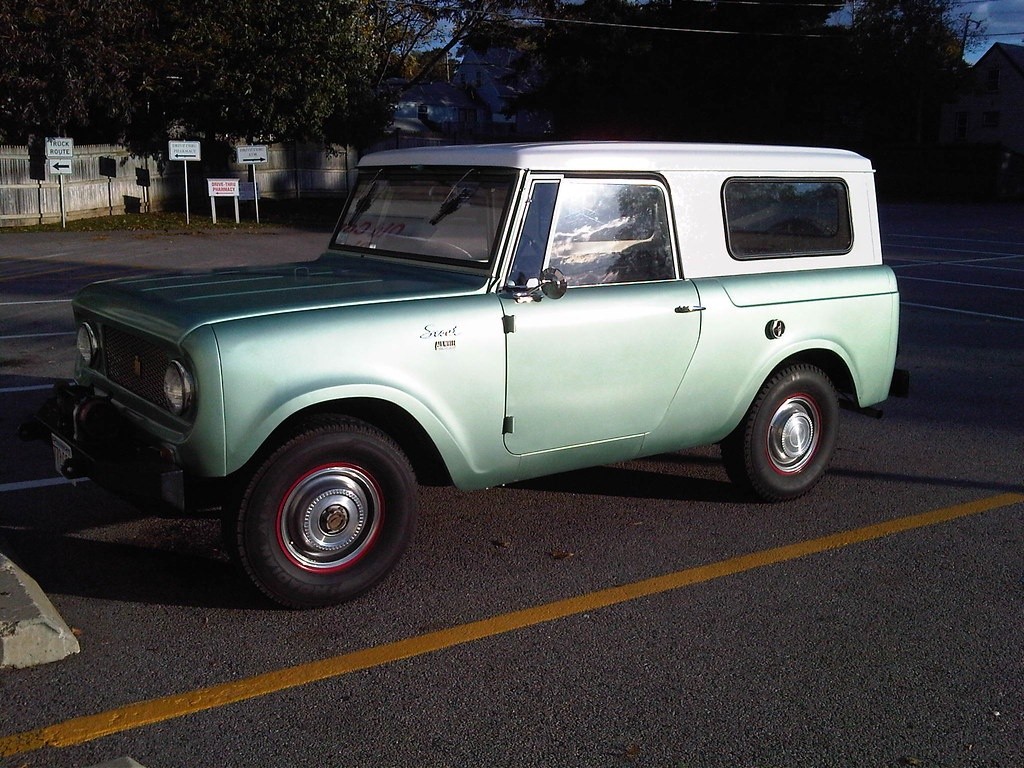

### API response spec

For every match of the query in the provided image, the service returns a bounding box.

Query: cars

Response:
[47,141,899,611]
